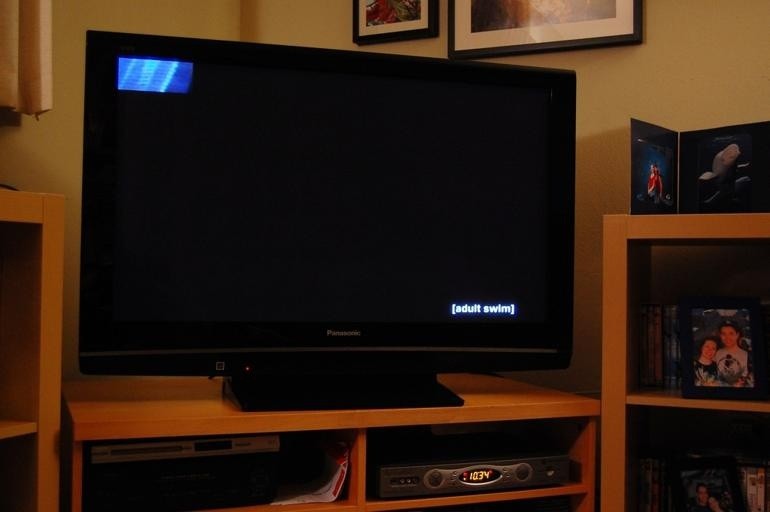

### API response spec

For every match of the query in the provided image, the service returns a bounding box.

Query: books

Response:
[734,459,769,510]
[629,454,680,510]
[634,303,689,393]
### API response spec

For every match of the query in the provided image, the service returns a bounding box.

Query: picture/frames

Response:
[448,0,642,60]
[353,0,439,46]
[678,296,770,400]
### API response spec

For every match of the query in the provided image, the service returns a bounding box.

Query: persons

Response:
[647,162,664,213]
[688,482,729,512]
[694,321,754,388]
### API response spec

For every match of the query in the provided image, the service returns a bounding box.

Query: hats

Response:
[719,317,739,332]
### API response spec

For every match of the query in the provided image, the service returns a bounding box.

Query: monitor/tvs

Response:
[78,28,577,411]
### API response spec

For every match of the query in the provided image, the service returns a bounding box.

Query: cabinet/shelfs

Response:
[600,214,770,512]
[0,190,65,512]
[61,367,600,512]
